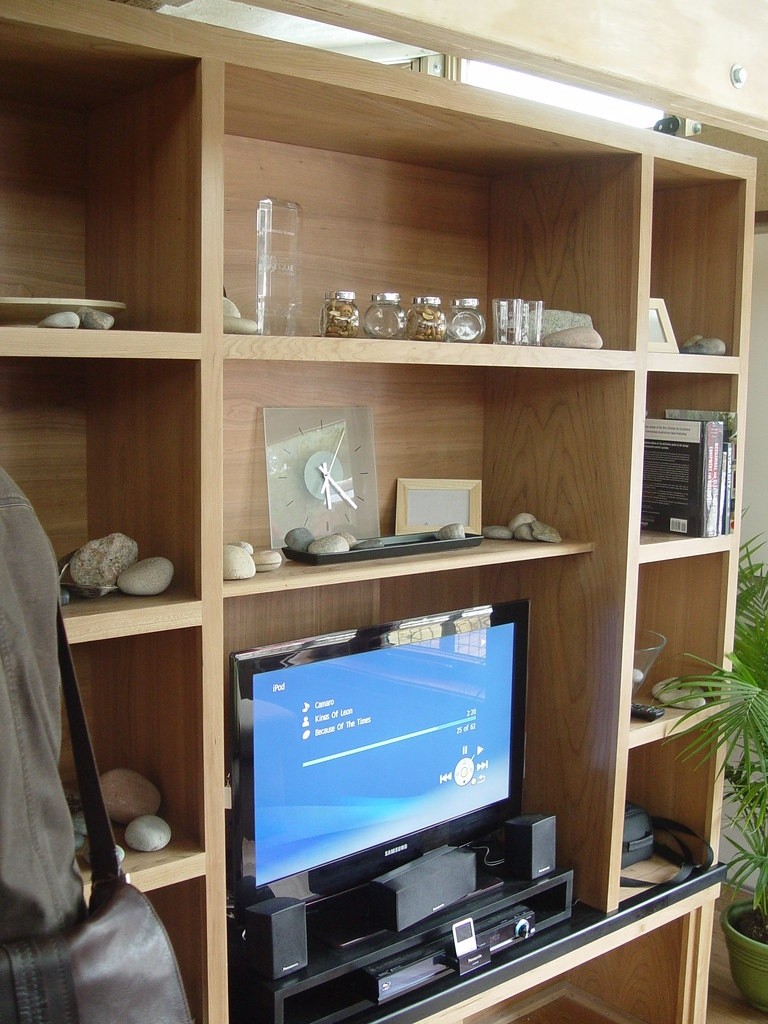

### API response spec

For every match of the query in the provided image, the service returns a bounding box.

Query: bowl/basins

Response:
[632,628,667,700]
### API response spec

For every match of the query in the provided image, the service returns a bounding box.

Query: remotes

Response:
[631,703,665,722]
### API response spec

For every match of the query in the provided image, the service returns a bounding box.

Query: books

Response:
[641,409,738,538]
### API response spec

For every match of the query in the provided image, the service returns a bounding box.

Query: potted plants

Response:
[648,504,768,1013]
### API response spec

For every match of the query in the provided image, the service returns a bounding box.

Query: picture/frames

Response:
[395,477,483,535]
[647,298,680,354]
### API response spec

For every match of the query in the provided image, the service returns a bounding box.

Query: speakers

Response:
[243,897,308,980]
[371,848,478,932]
[503,813,558,882]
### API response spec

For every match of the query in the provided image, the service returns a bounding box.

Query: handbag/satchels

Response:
[1,876,193,1024]
[619,801,714,912]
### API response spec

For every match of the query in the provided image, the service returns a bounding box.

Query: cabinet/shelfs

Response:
[0,0,762,1023]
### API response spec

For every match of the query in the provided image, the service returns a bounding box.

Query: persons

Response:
[0,468,87,947]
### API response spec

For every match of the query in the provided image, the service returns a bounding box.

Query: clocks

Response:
[263,407,381,550]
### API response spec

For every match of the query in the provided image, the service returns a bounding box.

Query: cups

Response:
[523,301,545,346]
[491,298,524,345]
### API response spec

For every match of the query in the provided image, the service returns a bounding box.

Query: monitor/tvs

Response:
[226,597,533,948]
